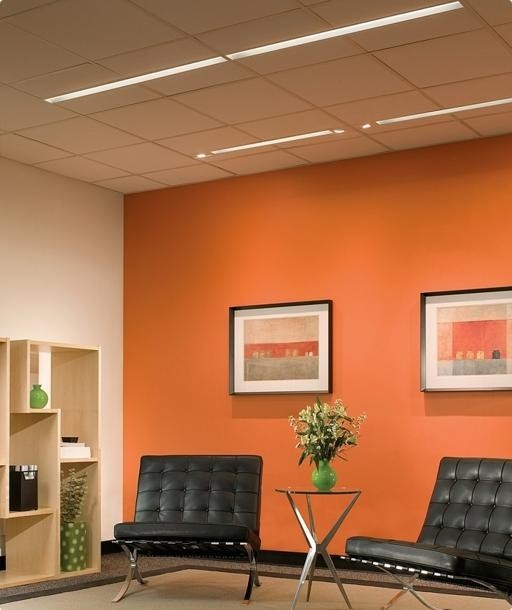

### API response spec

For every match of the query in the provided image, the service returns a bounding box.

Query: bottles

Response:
[30,383,48,408]
[312,458,337,489]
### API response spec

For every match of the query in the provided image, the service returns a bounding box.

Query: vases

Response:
[30,380,48,411]
[60,521,88,572]
[310,456,336,492]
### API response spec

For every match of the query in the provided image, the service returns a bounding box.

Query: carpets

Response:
[2,564,511,608]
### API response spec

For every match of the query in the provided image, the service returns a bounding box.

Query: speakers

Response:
[12,462,39,510]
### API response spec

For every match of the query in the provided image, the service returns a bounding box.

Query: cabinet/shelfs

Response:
[0,337,105,591]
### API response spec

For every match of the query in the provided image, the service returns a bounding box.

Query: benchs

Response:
[110,452,265,605]
[336,456,512,609]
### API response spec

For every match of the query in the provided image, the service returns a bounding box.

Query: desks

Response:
[273,488,361,609]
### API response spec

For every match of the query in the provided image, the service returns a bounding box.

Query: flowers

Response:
[59,468,89,525]
[287,397,369,469]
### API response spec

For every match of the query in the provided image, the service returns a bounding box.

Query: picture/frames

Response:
[418,285,512,394]
[227,300,335,397]
